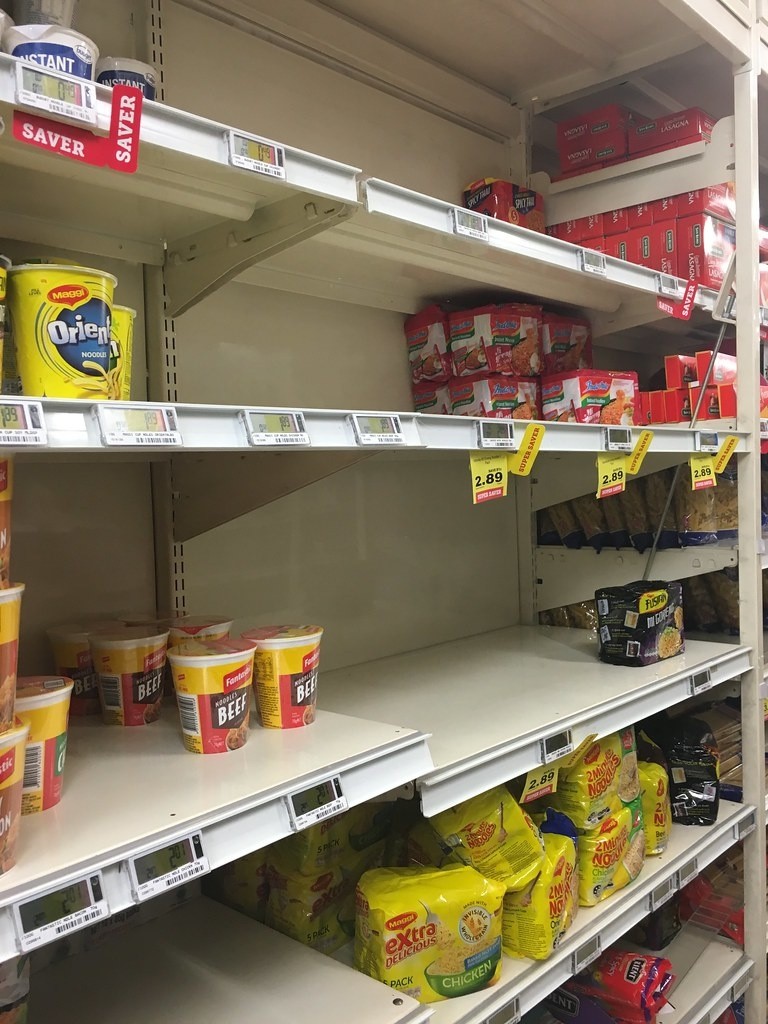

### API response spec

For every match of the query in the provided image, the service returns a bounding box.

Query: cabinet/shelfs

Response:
[156,0,768,1024]
[0,0,434,1024]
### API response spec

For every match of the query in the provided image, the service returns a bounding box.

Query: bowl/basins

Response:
[618,751,638,804]
[423,937,500,998]
[338,904,356,936]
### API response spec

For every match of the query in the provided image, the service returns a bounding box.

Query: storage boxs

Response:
[545,106,767,421]
